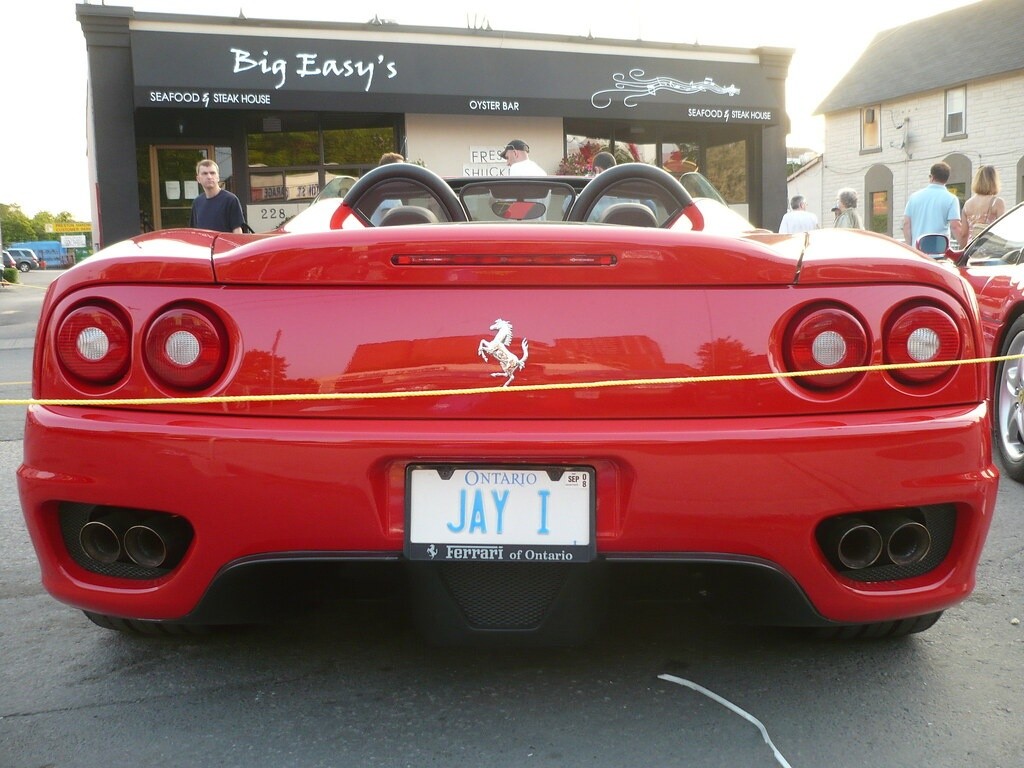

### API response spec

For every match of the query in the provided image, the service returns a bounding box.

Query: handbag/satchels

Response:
[972,197,996,240]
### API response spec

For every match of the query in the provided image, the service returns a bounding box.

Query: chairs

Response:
[564,161,706,231]
[340,163,471,230]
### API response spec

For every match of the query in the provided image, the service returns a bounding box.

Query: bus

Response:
[5,240,68,269]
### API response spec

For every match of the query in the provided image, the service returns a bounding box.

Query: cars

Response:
[901,195,1024,489]
[1,249,16,271]
[4,248,40,273]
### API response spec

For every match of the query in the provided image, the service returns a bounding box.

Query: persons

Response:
[779,195,819,234]
[189,159,246,234]
[561,152,641,222]
[489,141,553,221]
[903,161,962,258]
[370,152,406,227]
[831,188,863,230]
[959,165,1007,249]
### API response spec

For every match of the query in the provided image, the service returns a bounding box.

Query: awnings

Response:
[130,29,781,127]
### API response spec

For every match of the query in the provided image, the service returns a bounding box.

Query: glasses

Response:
[929,175,931,177]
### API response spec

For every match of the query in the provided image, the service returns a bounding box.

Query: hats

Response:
[831,206,838,211]
[500,140,529,159]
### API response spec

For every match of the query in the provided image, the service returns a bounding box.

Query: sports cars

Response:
[14,156,1001,640]
